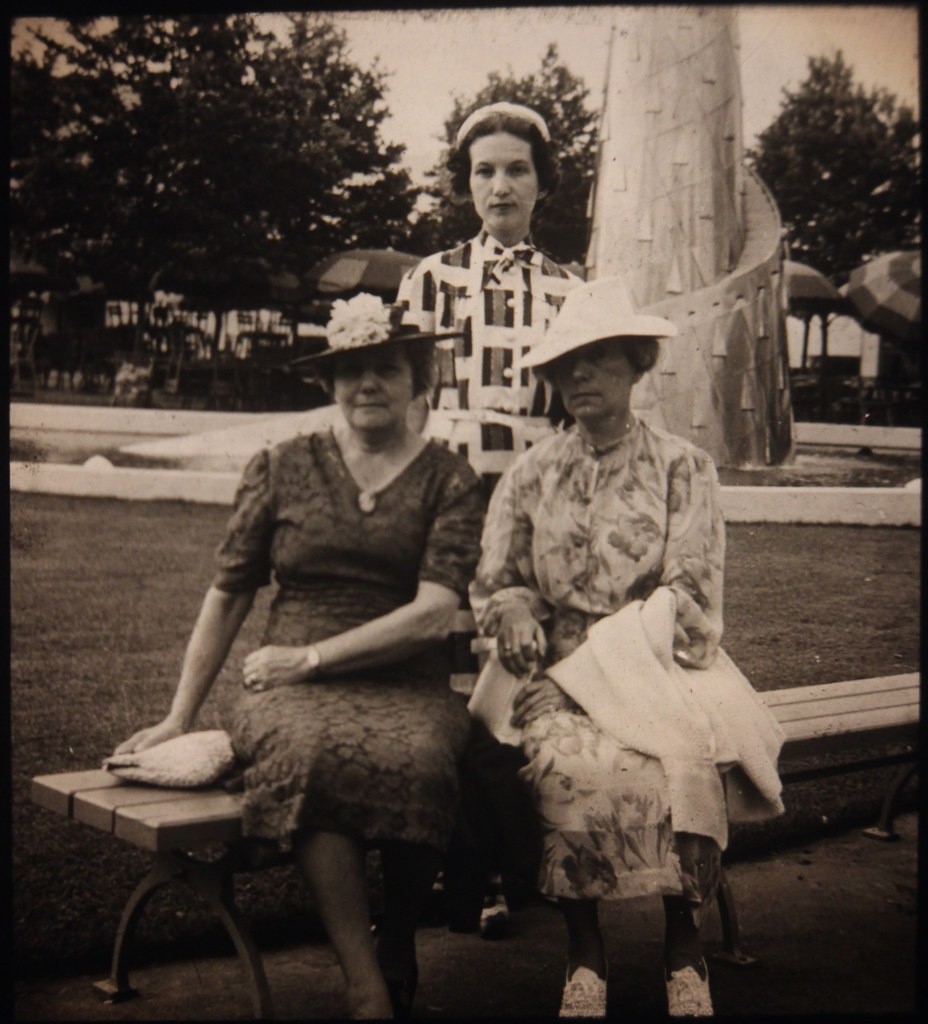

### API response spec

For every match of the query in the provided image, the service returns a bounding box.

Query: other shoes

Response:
[479,900,510,936]
[662,954,714,1018]
[557,957,609,1019]
[380,960,417,1017]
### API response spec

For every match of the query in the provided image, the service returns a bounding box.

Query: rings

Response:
[504,644,512,651]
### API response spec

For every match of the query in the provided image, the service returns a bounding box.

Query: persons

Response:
[396,103,587,939]
[469,280,723,1017]
[113,292,488,1024]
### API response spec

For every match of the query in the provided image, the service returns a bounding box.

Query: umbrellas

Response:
[744,251,924,355]
[150,246,430,298]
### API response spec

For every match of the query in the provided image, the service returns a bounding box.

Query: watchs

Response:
[304,644,324,675]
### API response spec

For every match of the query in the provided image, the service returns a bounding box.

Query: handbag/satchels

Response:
[466,647,537,746]
[102,729,234,788]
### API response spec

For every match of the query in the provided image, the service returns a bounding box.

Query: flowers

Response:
[324,288,394,350]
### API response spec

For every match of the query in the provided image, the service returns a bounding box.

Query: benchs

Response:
[35,676,920,1024]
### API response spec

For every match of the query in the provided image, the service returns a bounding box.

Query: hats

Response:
[518,276,677,368]
[289,293,465,365]
